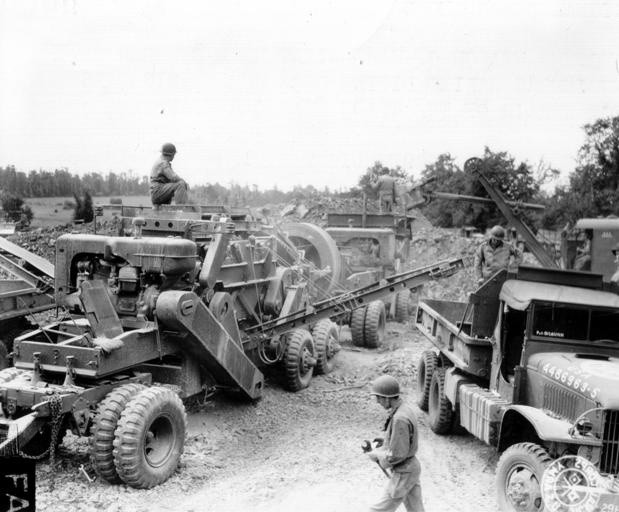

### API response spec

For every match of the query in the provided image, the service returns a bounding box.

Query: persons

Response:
[151,143,189,203]
[576,230,591,269]
[367,375,426,512]
[371,167,396,212]
[475,225,523,286]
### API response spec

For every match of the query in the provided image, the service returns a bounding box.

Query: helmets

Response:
[159,143,178,155]
[490,224,505,240]
[369,375,402,398]
